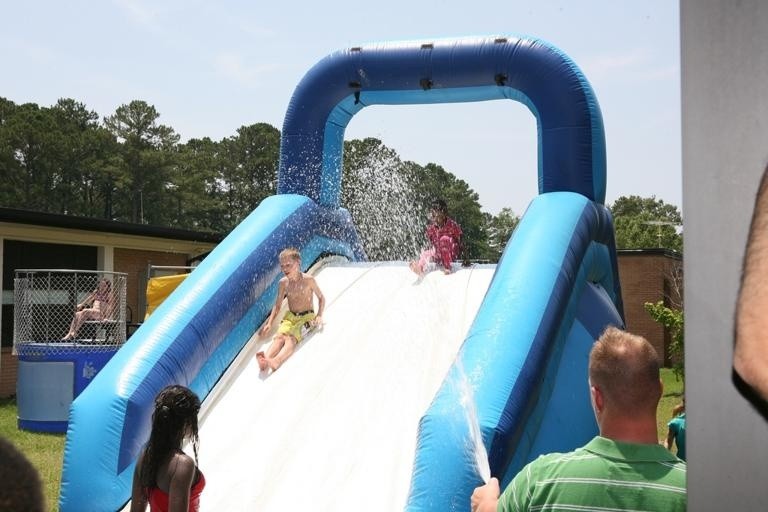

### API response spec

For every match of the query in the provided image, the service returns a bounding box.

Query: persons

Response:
[729,160,767,414]
[468,319,687,512]
[254,248,327,373]
[666,401,687,456]
[407,199,463,279]
[60,276,115,341]
[129,382,207,511]
